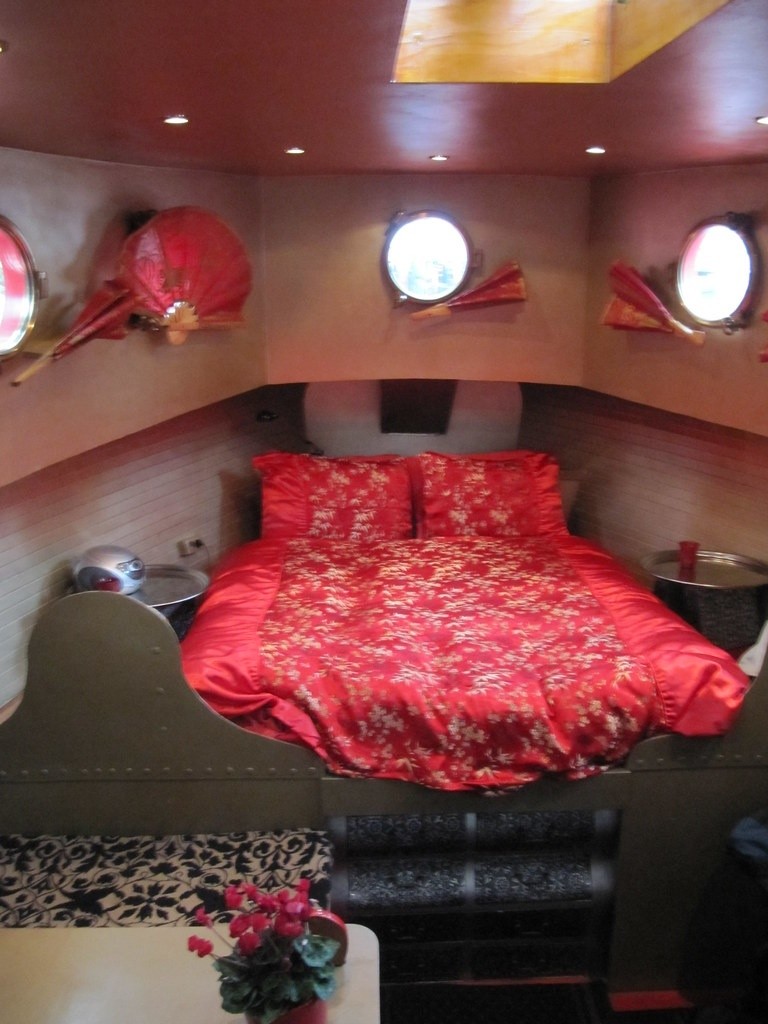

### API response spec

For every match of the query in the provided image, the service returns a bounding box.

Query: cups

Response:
[679,542,700,567]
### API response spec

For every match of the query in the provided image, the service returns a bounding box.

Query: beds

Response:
[0,536,768,992]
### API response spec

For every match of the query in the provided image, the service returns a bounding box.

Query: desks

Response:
[636,549,768,657]
[67,564,211,641]
[0,920,381,1024]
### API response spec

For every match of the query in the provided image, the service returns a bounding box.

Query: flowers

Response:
[187,877,341,1024]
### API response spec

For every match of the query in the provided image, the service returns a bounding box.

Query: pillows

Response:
[417,451,572,539]
[252,452,412,540]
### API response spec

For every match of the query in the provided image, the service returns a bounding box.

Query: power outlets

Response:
[183,538,202,556]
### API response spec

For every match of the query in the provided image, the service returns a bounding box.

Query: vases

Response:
[273,996,328,1024]
